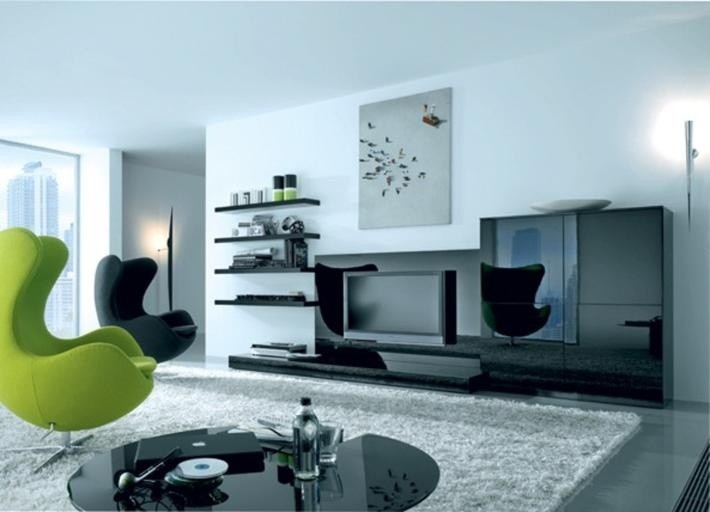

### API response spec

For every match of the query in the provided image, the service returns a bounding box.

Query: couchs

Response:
[316,263,378,339]
[94,255,196,363]
[479,263,552,350]
[0,228,156,474]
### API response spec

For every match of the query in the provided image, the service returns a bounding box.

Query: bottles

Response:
[294,480,321,512]
[292,397,319,480]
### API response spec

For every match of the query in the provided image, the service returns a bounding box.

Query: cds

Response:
[164,456,229,496]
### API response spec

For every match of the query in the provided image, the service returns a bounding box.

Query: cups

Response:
[316,421,343,464]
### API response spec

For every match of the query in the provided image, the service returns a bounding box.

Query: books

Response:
[230,254,271,269]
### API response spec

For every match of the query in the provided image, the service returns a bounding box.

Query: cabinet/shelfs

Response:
[228,349,482,394]
[214,197,320,308]
[479,205,676,407]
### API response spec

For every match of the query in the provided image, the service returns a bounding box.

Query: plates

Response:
[529,198,612,212]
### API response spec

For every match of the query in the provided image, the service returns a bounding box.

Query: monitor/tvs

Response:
[342,269,447,346]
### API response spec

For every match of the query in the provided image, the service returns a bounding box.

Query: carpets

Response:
[451,336,661,389]
[0,362,640,512]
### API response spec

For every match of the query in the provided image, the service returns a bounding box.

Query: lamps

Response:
[685,120,698,227]
[156,206,174,312]
